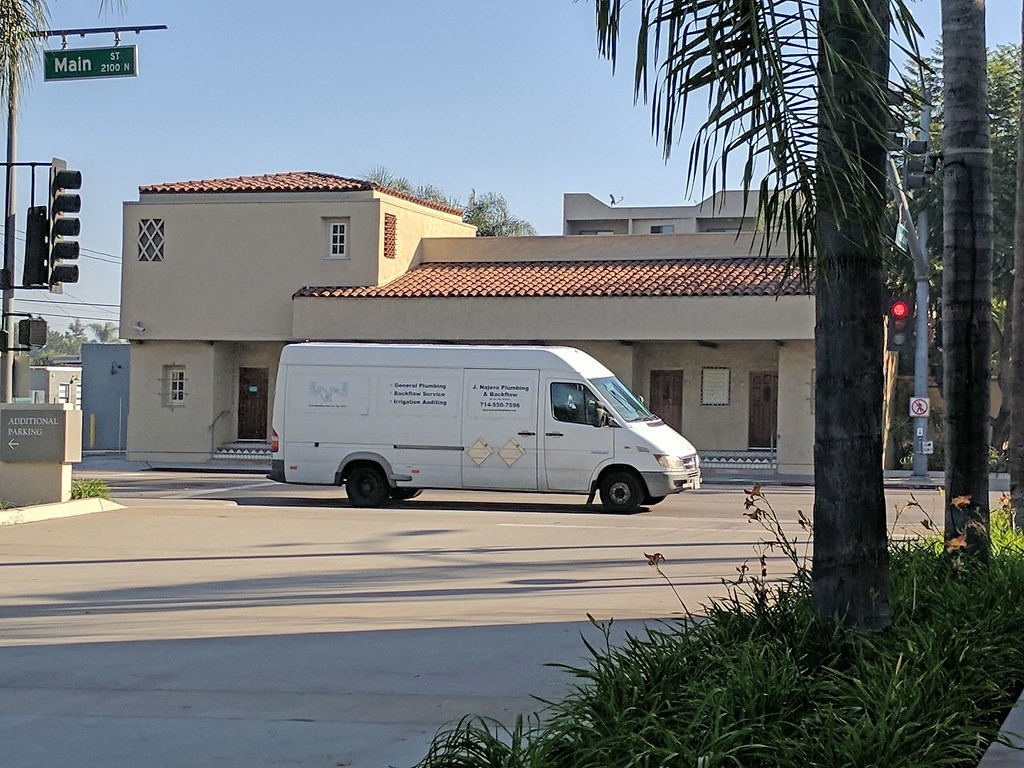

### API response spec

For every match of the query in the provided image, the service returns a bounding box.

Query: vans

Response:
[266,344,704,517]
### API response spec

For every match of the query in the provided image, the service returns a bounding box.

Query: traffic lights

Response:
[43,158,83,296]
[886,89,906,153]
[18,318,47,349]
[904,138,927,193]
[888,297,915,352]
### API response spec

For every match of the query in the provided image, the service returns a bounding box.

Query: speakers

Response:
[17,319,48,347]
